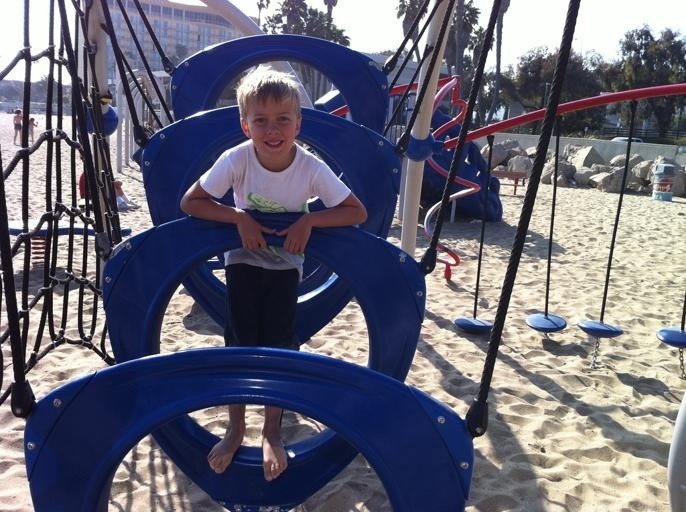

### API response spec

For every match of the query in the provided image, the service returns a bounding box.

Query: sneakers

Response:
[117,200,142,212]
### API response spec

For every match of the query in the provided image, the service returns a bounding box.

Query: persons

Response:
[80,172,141,211]
[28,118,38,145]
[14,110,22,145]
[180,66,367,481]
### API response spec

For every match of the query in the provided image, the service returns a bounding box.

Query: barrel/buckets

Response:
[652,163,675,200]
